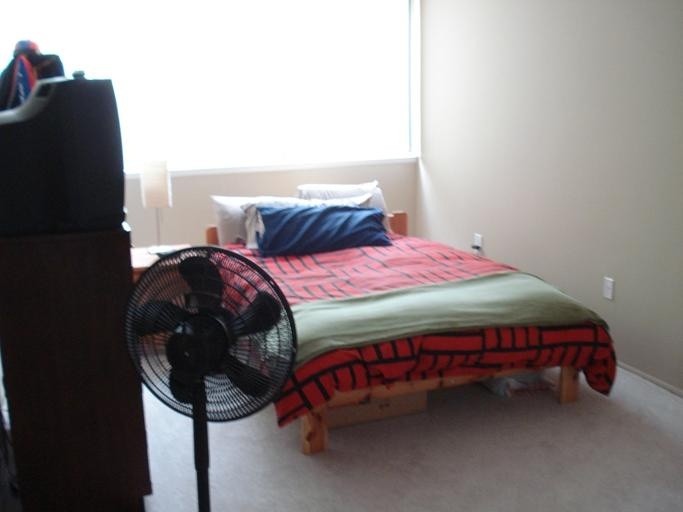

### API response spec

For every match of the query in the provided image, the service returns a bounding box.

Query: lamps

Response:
[140,160,174,255]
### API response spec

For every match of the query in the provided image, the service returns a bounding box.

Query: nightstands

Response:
[128,244,198,355]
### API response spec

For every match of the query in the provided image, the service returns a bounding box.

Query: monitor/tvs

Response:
[0,72,152,238]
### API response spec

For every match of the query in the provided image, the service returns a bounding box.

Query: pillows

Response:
[210,178,394,259]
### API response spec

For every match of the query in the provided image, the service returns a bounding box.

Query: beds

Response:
[206,211,607,455]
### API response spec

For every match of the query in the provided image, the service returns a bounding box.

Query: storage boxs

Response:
[324,388,427,431]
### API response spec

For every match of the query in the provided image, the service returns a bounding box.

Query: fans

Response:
[123,245,298,512]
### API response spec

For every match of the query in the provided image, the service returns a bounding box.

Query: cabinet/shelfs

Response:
[0,226,153,511]
